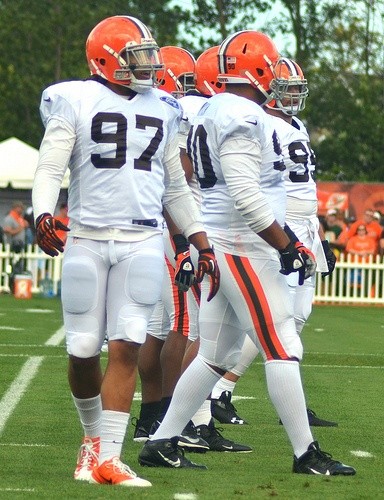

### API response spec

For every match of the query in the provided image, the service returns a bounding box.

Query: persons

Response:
[0,15,384,486]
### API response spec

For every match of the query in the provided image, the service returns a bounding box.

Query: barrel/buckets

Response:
[14,273,32,300]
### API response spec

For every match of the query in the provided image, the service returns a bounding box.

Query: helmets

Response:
[85,15,153,93]
[195,47,226,96]
[152,46,196,95]
[217,31,289,109]
[266,57,309,116]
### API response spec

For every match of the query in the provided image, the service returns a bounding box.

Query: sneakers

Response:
[89,456,152,487]
[279,409,338,427]
[137,438,207,469]
[195,417,252,453]
[134,416,209,450]
[74,436,100,480]
[206,391,245,424]
[292,441,357,476]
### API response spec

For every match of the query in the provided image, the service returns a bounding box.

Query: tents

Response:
[0,138,71,190]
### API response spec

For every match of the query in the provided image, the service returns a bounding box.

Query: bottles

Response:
[39,272,53,299]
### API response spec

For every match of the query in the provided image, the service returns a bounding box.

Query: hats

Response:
[13,201,24,209]
[327,209,337,215]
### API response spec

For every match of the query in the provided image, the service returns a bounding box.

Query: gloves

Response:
[295,242,317,280]
[36,212,70,257]
[197,245,221,302]
[173,242,194,291]
[279,243,305,285]
[320,240,337,277]
